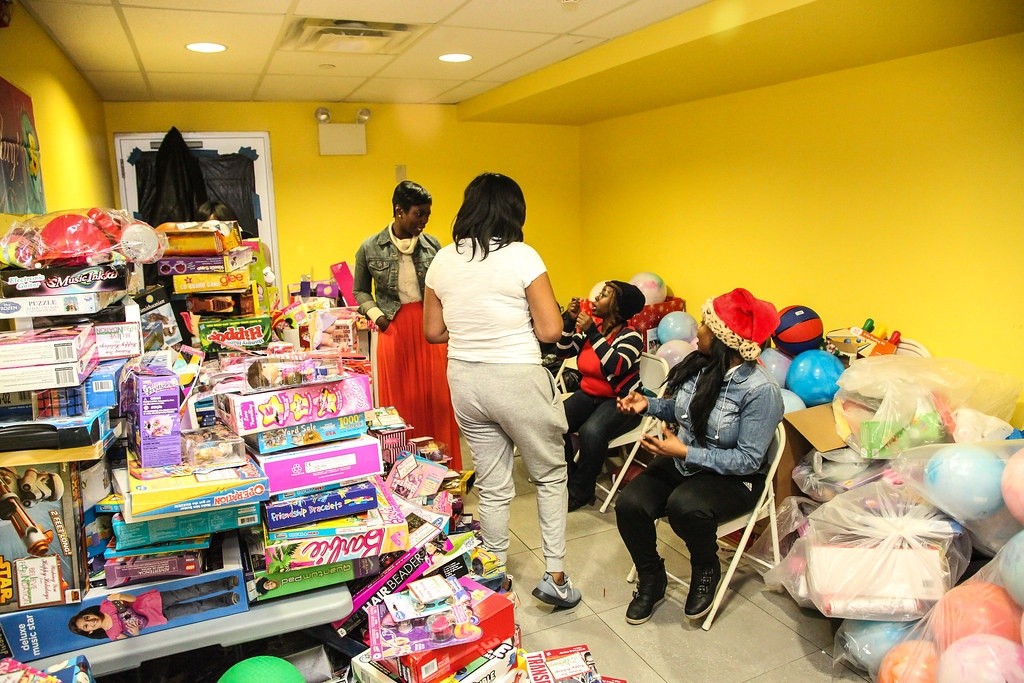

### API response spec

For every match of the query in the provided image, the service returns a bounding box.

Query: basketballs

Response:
[771,305,824,354]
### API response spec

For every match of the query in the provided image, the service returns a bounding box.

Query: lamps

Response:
[314,105,371,156]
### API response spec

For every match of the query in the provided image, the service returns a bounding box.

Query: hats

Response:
[701,287,781,363]
[605,278,647,316]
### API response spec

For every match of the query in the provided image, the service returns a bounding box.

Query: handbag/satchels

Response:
[741,350,1024,683]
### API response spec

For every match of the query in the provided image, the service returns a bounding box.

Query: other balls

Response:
[763,347,846,406]
[878,443,1024,683]
[217,655,305,683]
[588,273,698,367]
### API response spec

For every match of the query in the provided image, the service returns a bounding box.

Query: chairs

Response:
[627,420,787,632]
[514,356,579,483]
[574,352,671,513]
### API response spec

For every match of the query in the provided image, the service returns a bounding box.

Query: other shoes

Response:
[566,495,594,512]
[683,557,722,619]
[503,574,516,596]
[531,573,580,608]
[625,558,668,625]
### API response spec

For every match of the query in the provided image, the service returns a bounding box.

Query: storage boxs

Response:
[0,220,631,683]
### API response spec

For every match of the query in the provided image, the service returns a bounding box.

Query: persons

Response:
[421,172,581,609]
[68,575,242,641]
[616,288,785,624]
[246,577,279,601]
[353,181,480,533]
[247,357,343,392]
[562,280,646,513]
